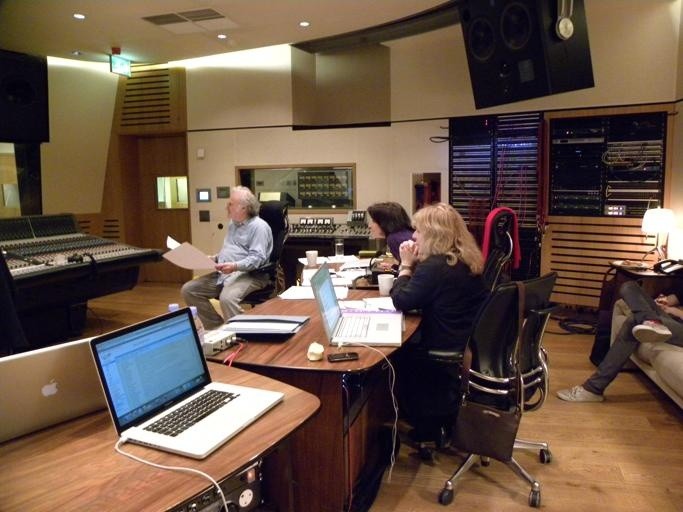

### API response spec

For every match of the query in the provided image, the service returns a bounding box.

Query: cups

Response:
[378,274,397,295]
[335,239,344,257]
[306,251,318,267]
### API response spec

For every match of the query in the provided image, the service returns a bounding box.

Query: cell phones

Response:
[327,351,359,363]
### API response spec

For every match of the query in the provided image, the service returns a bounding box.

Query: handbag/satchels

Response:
[442,278,527,463]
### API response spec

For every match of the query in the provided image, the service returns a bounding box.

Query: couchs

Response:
[610,294,683,407]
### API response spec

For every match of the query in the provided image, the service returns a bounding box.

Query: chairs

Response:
[484,209,549,363]
[239,199,291,307]
[417,270,560,509]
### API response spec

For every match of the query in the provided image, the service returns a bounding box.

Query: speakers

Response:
[455,1,596,111]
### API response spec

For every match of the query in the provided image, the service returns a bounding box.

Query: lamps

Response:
[640,207,676,261]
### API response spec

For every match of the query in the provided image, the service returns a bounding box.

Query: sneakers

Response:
[555,383,606,403]
[631,321,673,344]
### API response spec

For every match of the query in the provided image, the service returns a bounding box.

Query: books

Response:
[223,314,310,335]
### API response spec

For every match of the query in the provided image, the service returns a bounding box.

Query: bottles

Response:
[190,306,206,360]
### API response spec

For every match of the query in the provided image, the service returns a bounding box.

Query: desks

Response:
[616,267,674,293]
[288,235,370,255]
[0,249,165,353]
[0,359,322,508]
[300,256,396,288]
[206,288,424,512]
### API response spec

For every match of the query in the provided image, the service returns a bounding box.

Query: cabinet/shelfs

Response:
[211,361,384,511]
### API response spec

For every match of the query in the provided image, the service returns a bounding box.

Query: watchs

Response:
[398,263,413,272]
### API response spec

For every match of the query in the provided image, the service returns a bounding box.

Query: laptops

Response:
[311,261,402,350]
[87,307,284,462]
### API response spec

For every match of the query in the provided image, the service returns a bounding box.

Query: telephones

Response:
[651,259,683,278]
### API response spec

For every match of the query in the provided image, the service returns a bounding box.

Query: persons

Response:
[555,275,683,403]
[180,185,274,331]
[368,202,416,270]
[363,201,485,448]
